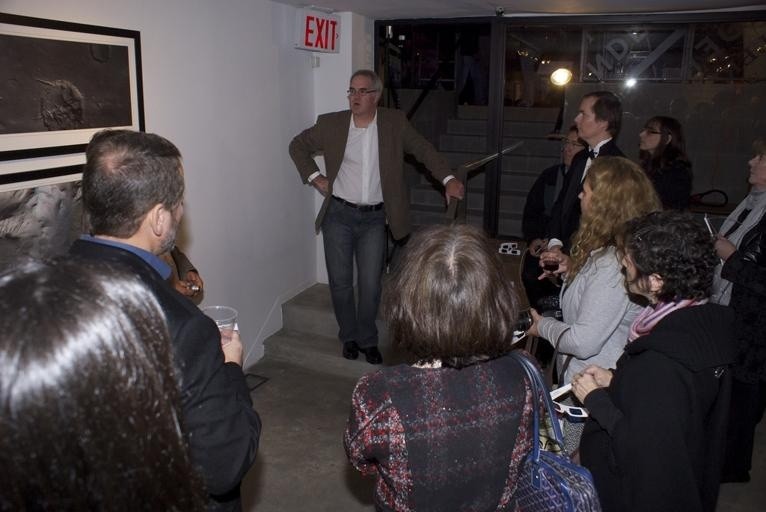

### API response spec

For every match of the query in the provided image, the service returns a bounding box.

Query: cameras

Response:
[515,295,563,331]
[181,279,200,291]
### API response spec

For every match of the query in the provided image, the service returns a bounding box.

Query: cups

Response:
[200,305,240,346]
[542,250,561,272]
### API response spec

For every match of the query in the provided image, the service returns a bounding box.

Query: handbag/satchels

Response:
[505,449,601,511]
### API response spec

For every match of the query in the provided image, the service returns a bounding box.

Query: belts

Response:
[331,194,384,212]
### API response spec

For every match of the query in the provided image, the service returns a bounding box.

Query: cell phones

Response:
[535,245,542,255]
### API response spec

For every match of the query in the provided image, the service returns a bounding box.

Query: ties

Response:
[588,148,597,162]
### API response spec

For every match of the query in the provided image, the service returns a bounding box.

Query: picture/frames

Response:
[0,13,146,161]
[0,164,89,278]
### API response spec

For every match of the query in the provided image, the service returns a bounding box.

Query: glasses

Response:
[561,137,581,150]
[347,87,377,96]
[644,126,668,138]
